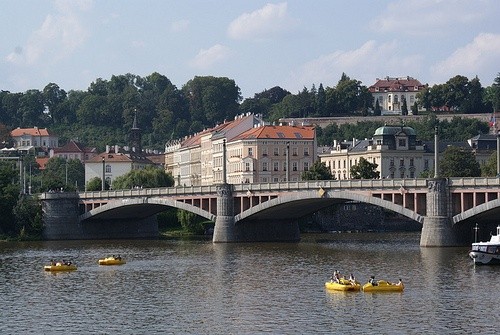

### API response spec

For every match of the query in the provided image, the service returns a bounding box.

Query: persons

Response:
[329,270,356,286]
[113,254,122,260]
[50,260,72,266]
[369,276,402,287]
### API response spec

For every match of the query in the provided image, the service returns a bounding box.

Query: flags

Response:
[489,112,496,126]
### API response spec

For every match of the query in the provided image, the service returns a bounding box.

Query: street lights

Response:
[286,141,291,181]
[434,125,439,178]
[496,129,500,177]
[19,154,25,194]
[222,136,226,183]
[101,156,105,191]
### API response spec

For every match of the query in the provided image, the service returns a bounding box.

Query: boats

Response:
[43,264,78,271]
[324,277,362,292]
[98,256,127,265]
[469,223,500,267]
[361,280,405,293]
